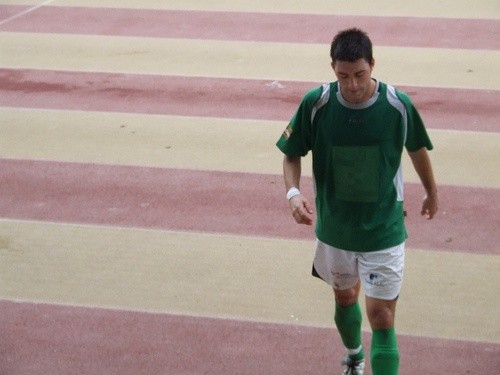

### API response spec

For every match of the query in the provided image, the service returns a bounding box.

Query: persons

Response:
[277,29,440,375]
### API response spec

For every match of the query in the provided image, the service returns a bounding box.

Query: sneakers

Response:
[341,352,367,375]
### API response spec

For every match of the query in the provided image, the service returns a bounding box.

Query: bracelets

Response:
[287,189,300,201]
